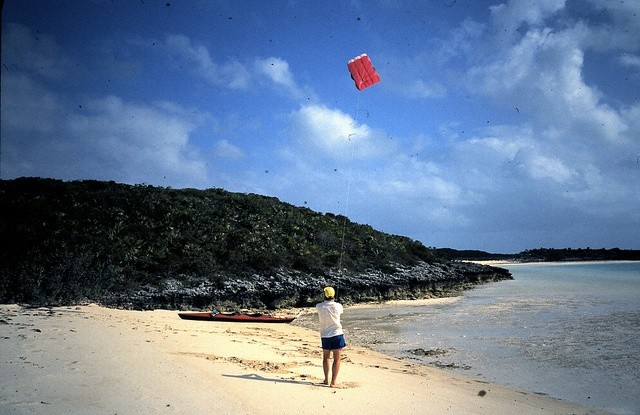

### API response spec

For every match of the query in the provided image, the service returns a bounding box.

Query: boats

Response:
[178,311,295,323]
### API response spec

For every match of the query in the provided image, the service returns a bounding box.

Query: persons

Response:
[315,286,345,388]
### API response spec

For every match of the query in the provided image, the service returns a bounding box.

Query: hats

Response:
[324,287,335,298]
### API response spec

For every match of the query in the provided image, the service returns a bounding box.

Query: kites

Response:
[346,53,380,91]
